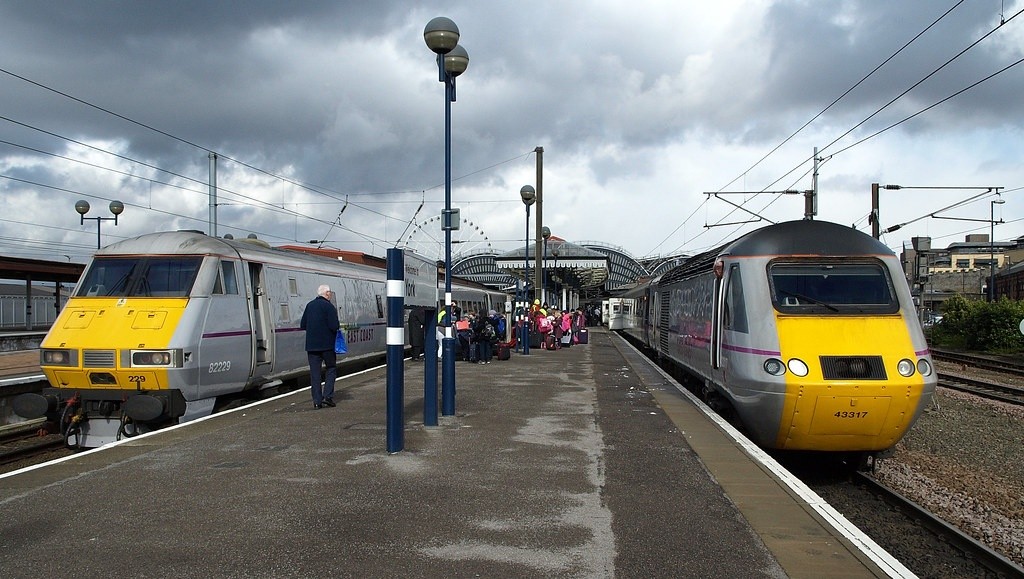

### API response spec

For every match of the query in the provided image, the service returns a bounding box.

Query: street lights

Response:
[520,184,536,317]
[1003,255,1012,300]
[542,226,552,302]
[551,247,560,310]
[421,14,472,415]
[74,199,124,250]
[989,198,1005,301]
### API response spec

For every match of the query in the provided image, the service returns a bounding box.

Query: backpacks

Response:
[481,319,496,340]
[494,317,505,334]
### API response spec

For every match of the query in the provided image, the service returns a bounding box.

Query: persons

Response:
[300,285,341,410]
[408,298,602,364]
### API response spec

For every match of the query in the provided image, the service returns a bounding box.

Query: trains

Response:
[12,227,533,451]
[596,218,939,473]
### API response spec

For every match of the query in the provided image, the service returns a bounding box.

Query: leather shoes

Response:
[321,396,336,407]
[314,403,323,410]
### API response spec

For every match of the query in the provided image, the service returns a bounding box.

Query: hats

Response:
[489,310,497,315]
[534,299,540,305]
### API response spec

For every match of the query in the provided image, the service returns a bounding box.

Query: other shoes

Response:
[412,357,421,361]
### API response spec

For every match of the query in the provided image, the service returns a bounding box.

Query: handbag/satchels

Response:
[334,329,347,354]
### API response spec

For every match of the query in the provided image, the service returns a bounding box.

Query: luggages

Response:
[561,336,571,348]
[497,343,511,360]
[469,344,479,363]
[546,336,556,351]
[578,328,588,344]
